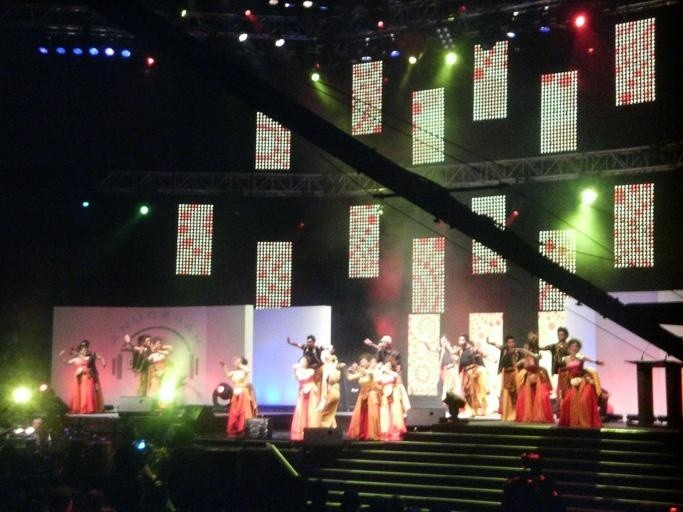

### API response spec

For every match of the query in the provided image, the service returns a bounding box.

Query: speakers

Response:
[406,407,445,431]
[165,422,195,444]
[117,395,160,416]
[304,427,345,447]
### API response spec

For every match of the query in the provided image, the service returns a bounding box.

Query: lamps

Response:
[439,392,469,425]
[212,383,233,413]
[30,0,618,70]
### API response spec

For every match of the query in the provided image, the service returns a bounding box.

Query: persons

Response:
[426,333,488,418]
[486,327,609,429]
[1,410,407,512]
[501,453,559,510]
[59,340,106,414]
[337,334,411,442]
[219,356,257,435]
[287,334,342,440]
[121,334,172,395]
